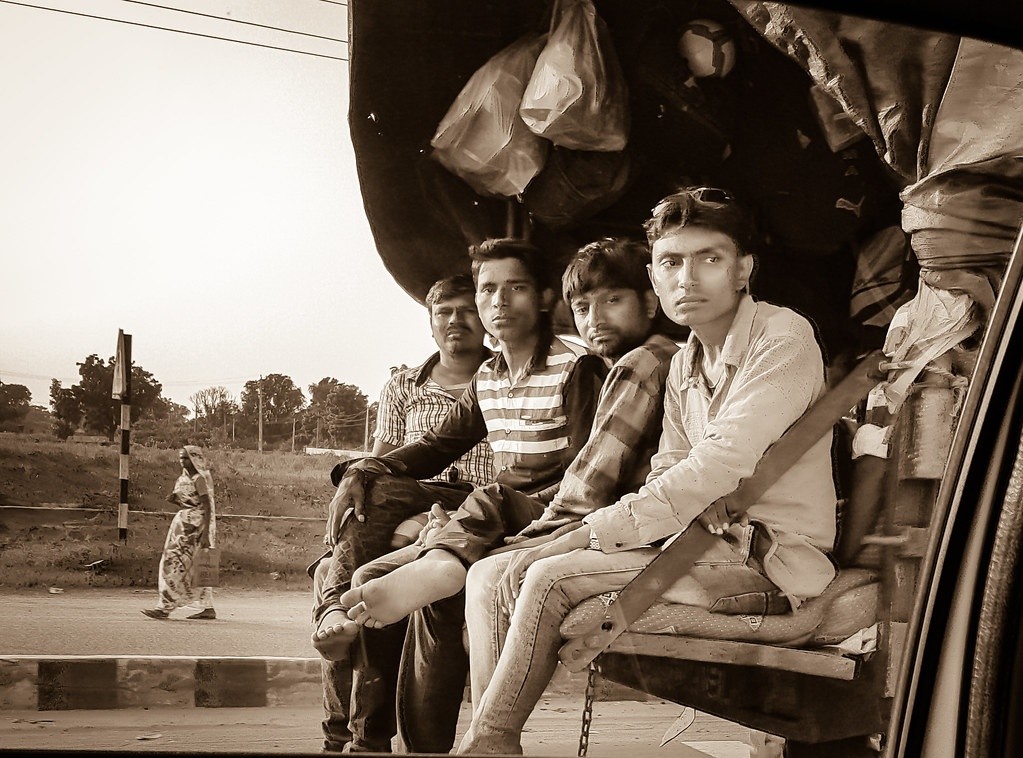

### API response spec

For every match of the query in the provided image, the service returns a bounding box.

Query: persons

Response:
[306,186,836,758]
[140,445,219,620]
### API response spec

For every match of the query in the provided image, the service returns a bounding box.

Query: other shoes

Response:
[184,612,215,620]
[140,609,169,620]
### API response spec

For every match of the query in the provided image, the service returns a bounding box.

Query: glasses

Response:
[649,188,734,220]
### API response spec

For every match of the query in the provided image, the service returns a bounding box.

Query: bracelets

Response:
[587,529,602,551]
[360,470,369,490]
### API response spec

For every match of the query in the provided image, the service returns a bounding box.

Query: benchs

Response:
[556,359,892,680]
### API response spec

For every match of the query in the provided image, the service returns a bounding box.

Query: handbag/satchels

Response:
[429,27,551,203]
[520,0,632,152]
[193,541,220,589]
